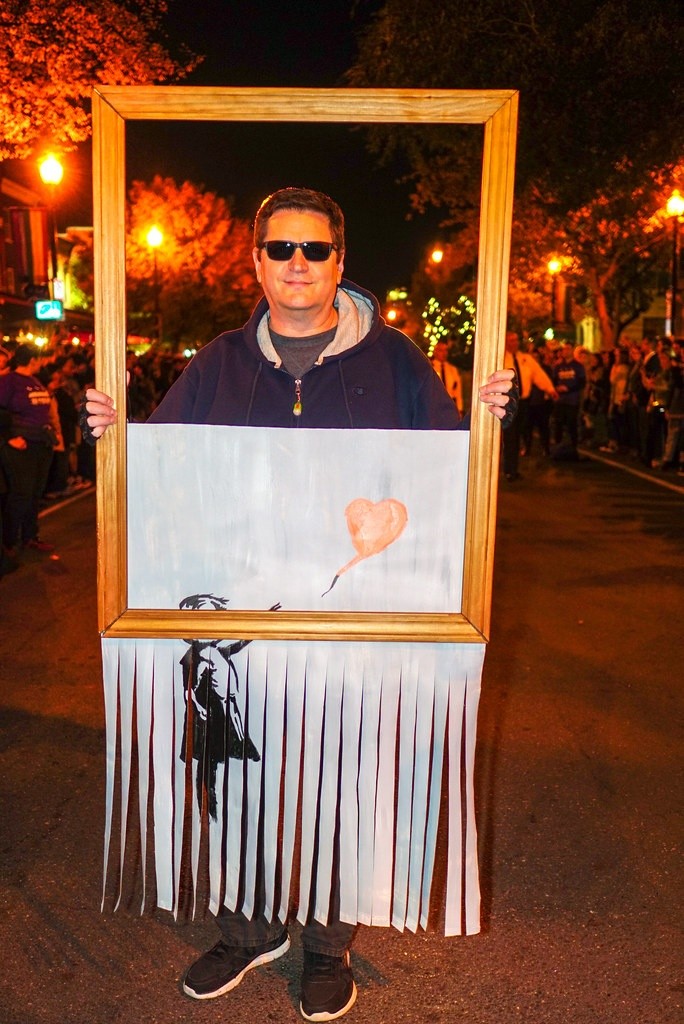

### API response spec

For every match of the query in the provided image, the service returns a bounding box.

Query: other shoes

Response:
[520,447,530,458]
[20,538,54,551]
[3,545,15,558]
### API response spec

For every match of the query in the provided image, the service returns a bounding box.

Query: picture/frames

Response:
[86,88,518,642]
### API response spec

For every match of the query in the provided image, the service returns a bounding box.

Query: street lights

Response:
[546,257,562,323]
[38,156,64,279]
[148,227,163,341]
[669,187,684,338]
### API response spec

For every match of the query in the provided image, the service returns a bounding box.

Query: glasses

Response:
[259,240,339,262]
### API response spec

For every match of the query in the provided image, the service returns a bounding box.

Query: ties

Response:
[513,354,522,399]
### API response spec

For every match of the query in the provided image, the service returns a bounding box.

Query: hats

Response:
[15,342,55,361]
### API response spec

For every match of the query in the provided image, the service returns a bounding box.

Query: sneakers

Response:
[183,933,291,999]
[299,944,357,1022]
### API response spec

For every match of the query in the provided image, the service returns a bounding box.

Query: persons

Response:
[429,328,684,479]
[0,334,188,570]
[86,185,515,1022]
